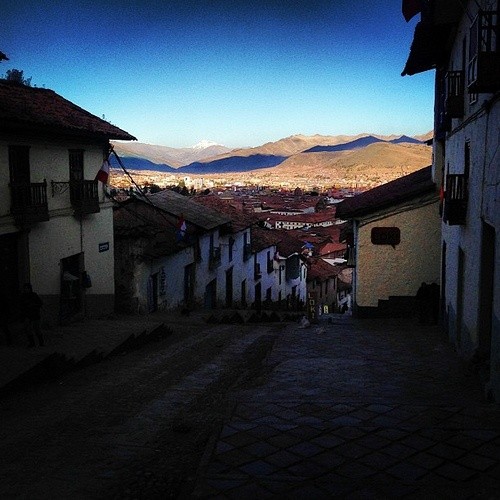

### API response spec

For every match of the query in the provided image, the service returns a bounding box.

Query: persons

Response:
[20,284,44,348]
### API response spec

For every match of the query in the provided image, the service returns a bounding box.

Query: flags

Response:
[175,212,186,241]
[96,143,114,184]
